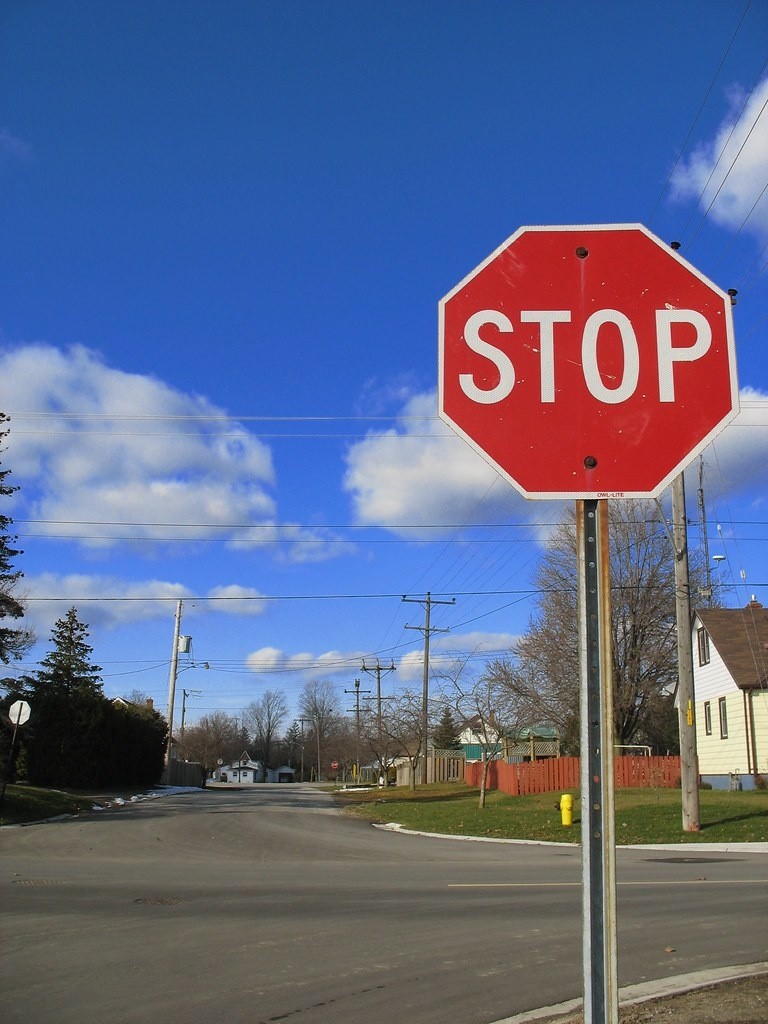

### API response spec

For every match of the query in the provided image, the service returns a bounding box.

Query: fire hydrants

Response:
[558,794,575,827]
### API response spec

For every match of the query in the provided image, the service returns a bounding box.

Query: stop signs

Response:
[432,222,741,506]
[332,761,339,769]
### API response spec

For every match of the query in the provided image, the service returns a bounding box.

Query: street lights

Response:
[163,662,210,787]
[704,554,727,609]
